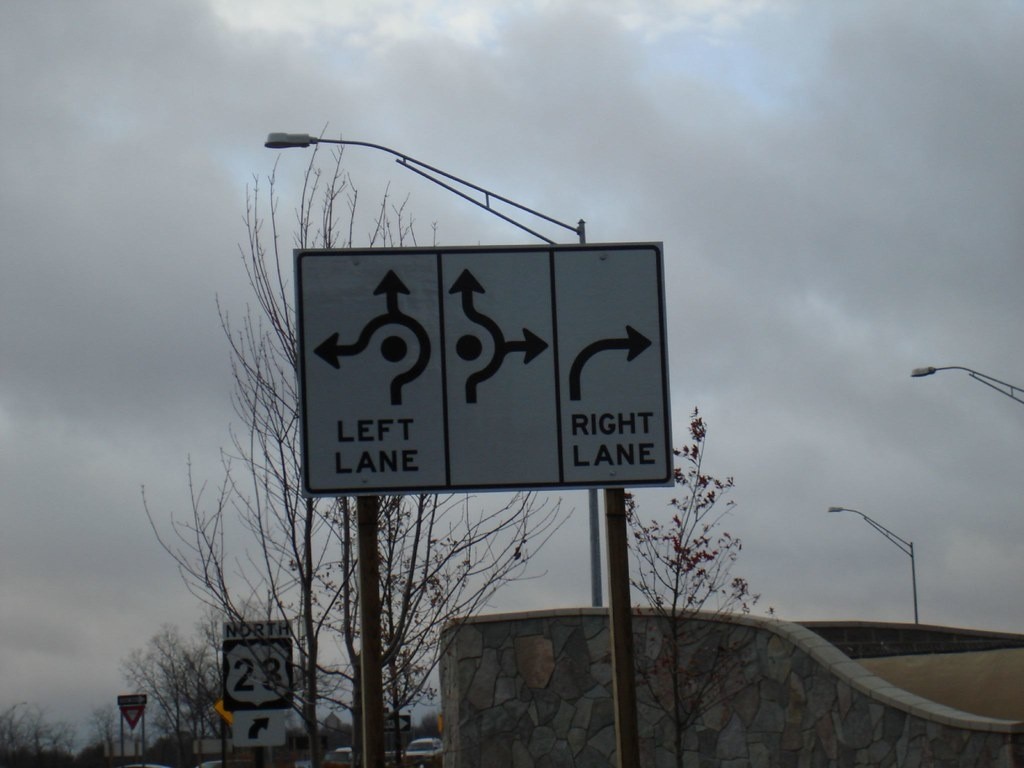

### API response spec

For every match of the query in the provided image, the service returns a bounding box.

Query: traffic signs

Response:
[384,716,411,733]
[291,246,676,496]
[222,622,294,748]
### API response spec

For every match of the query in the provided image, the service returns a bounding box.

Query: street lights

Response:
[828,506,919,625]
[264,131,641,768]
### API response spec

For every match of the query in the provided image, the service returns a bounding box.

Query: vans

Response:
[405,738,443,756]
